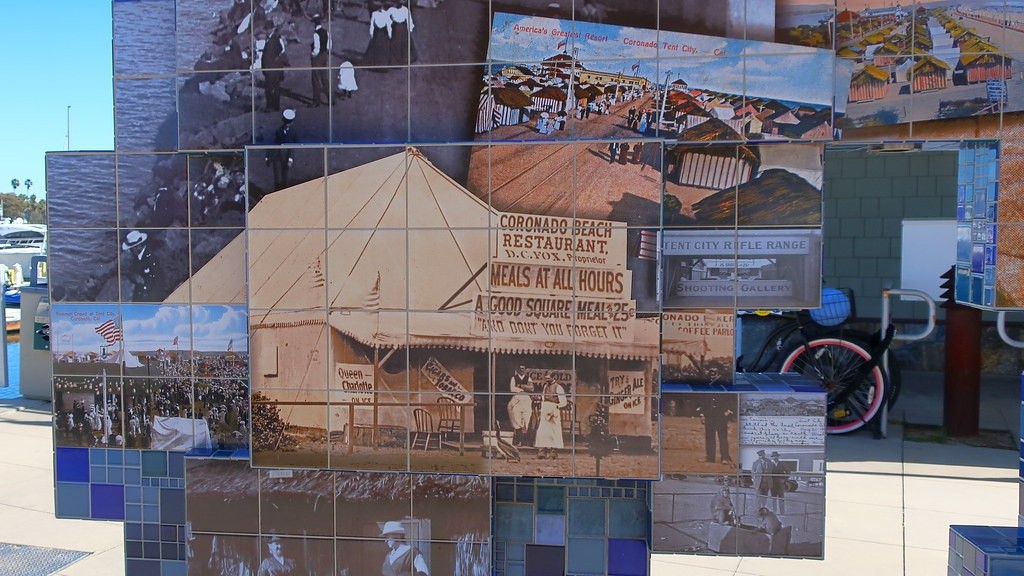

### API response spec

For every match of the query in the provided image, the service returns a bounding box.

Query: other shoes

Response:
[537,452,548,459]
[549,451,559,461]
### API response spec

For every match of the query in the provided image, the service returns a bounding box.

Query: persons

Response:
[657,349,738,419]
[119,229,166,302]
[537,1,671,171]
[378,519,430,576]
[694,360,735,465]
[535,372,567,460]
[766,450,791,517]
[51,308,249,459]
[507,362,535,446]
[751,450,774,515]
[148,1,449,237]
[254,534,298,576]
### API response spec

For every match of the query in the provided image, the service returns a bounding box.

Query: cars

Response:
[0,227,48,276]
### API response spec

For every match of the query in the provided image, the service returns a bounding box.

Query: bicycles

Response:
[737,276,890,439]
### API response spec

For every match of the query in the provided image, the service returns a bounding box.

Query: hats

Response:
[757,450,766,456]
[268,533,286,544]
[380,521,404,536]
[770,451,780,457]
